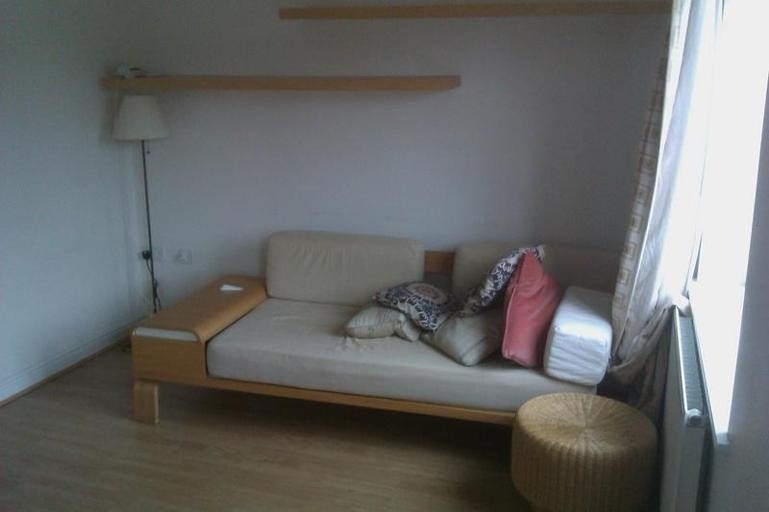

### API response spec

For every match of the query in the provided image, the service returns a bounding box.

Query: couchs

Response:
[129,232,620,428]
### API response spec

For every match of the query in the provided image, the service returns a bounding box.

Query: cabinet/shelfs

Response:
[101,0,672,94]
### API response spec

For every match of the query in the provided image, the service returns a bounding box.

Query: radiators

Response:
[661,303,712,512]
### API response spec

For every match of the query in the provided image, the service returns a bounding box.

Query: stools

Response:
[509,392,659,512]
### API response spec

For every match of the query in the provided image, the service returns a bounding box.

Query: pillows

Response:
[500,249,562,369]
[372,280,462,334]
[417,307,506,368]
[457,243,547,319]
[343,298,425,344]
[542,284,615,387]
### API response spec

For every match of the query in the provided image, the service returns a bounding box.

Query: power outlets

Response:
[136,241,160,262]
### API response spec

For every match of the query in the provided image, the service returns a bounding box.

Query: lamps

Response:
[114,93,174,314]
